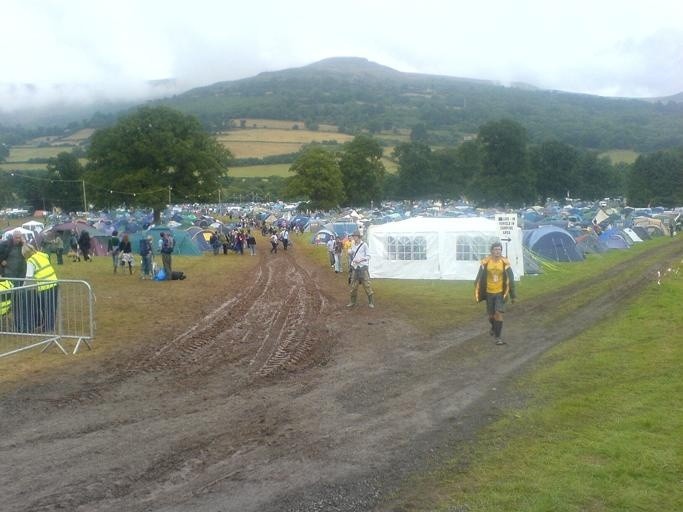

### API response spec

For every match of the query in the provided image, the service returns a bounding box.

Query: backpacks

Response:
[161,238,174,253]
[139,239,150,255]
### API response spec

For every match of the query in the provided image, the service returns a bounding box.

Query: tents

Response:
[2,201,681,279]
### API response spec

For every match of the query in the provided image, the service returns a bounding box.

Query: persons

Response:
[474,243,515,345]
[345,230,376,309]
[21,243,60,331]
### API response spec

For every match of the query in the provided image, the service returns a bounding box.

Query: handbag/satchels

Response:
[111,245,119,256]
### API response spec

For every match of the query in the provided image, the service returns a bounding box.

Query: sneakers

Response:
[496,336,502,344]
[368,303,374,309]
[346,302,354,307]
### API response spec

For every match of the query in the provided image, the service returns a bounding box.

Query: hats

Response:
[348,230,359,236]
